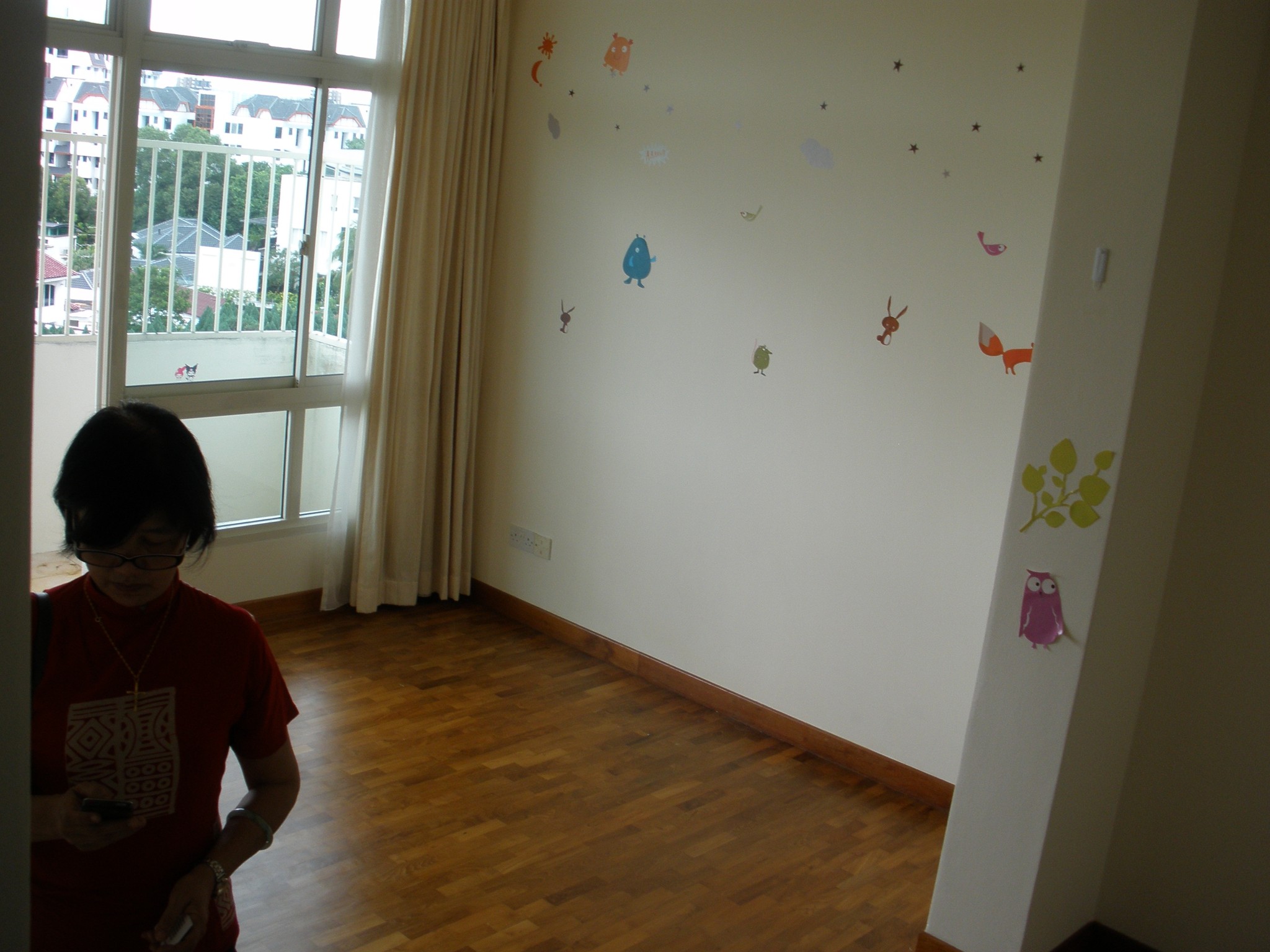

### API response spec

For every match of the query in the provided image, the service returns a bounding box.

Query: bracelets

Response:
[226,808,274,851]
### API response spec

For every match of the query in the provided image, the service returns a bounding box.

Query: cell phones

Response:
[80,797,135,822]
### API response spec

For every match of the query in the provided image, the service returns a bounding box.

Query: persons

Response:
[31,402,301,952]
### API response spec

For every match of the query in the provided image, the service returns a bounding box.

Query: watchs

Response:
[202,856,229,898]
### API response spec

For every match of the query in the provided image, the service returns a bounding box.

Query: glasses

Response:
[74,534,189,571]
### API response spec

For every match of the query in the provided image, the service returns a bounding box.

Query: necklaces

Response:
[84,573,174,711]
